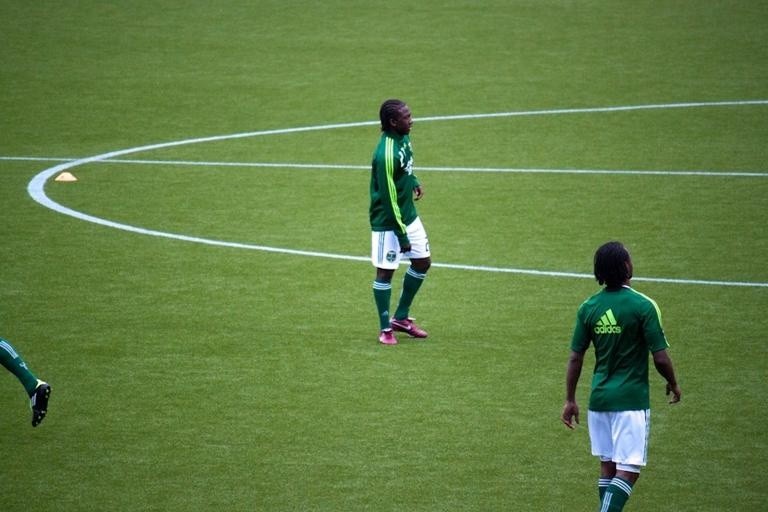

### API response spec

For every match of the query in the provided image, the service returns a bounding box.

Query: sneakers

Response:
[390,315,429,339]
[29,378,51,428]
[378,327,398,345]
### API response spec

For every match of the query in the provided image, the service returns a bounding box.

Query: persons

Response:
[560,242,681,512]
[0,337,51,427]
[369,100,431,345]
